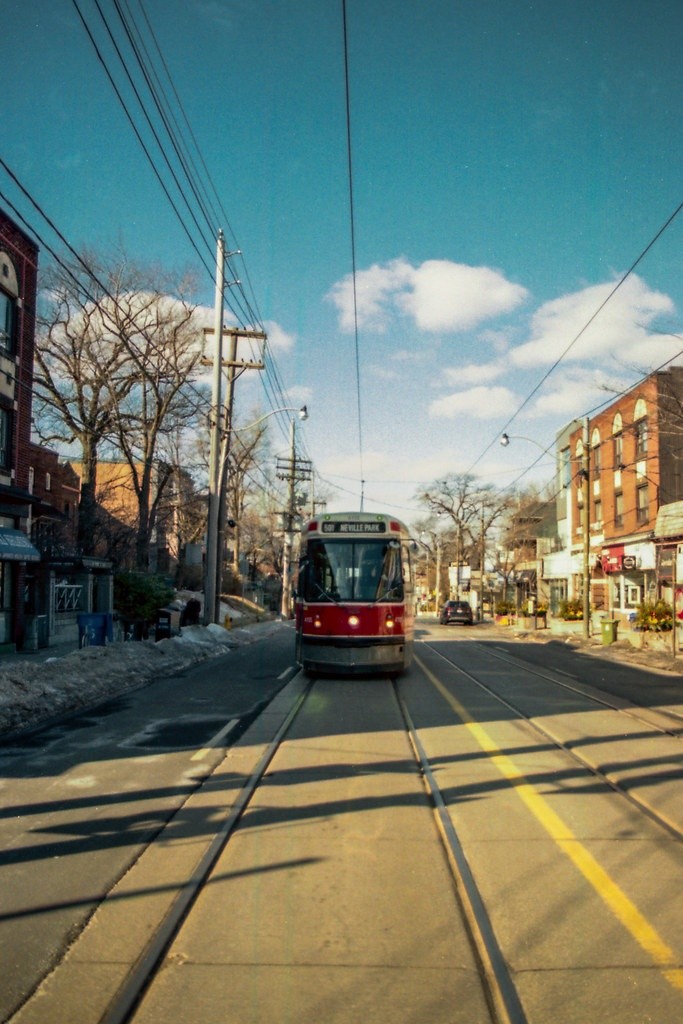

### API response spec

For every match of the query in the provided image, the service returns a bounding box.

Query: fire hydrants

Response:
[223,613,232,630]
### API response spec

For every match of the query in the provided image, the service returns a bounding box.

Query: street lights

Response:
[499,433,591,640]
[203,406,310,626]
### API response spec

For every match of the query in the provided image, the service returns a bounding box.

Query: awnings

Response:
[0,527,41,564]
[497,569,536,586]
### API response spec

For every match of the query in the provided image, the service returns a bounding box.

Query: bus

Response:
[292,512,418,679]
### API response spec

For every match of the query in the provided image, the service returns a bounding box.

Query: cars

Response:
[440,600,473,626]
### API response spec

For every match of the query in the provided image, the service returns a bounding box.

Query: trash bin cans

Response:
[600,619,620,646]
[155,606,182,643]
[75,611,115,651]
